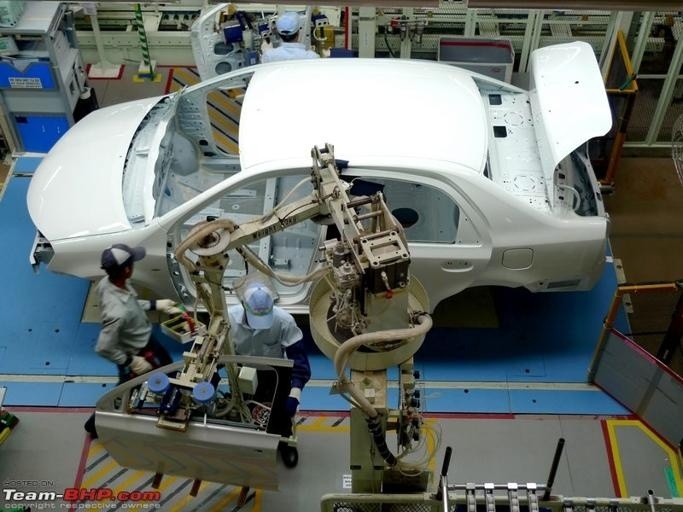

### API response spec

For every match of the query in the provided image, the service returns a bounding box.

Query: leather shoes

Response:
[280,437,298,468]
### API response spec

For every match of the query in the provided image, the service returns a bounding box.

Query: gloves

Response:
[285,387,301,416]
[154,298,183,315]
[123,355,154,377]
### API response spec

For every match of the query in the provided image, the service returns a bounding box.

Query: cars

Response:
[17,37,623,324]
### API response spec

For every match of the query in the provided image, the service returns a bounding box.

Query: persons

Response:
[256,10,321,66]
[83,243,183,437]
[205,281,312,470]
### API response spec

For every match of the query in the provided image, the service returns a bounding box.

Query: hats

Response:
[241,282,274,330]
[100,243,145,273]
